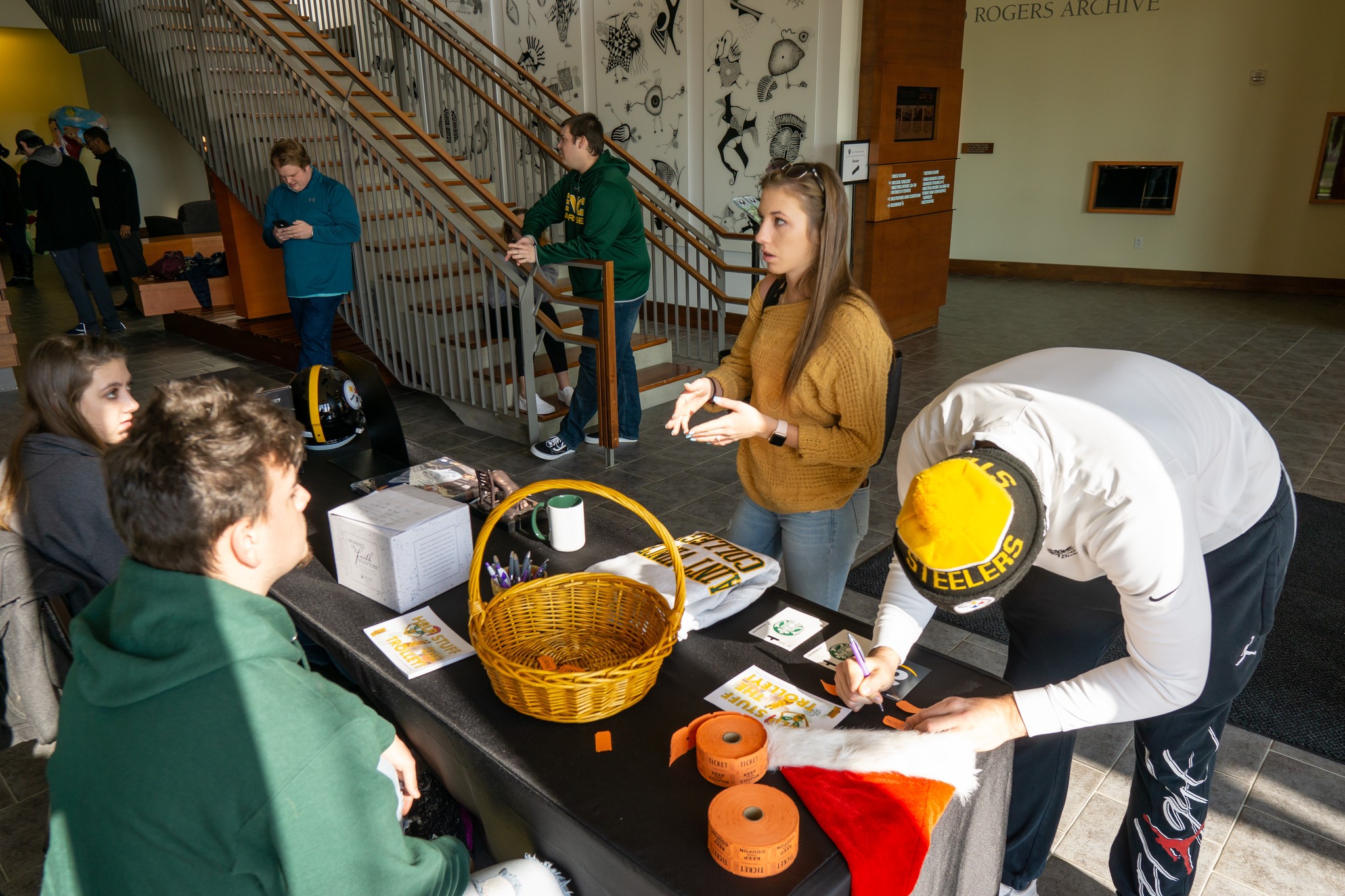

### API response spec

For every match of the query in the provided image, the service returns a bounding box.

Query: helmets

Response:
[290,362,365,450]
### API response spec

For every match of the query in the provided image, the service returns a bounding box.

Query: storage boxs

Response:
[325,483,474,612]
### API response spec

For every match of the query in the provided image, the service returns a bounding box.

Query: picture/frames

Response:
[1309,110,1345,206]
[1087,161,1185,216]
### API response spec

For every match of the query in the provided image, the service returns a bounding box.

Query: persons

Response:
[83,126,148,315]
[14,129,126,336]
[0,334,130,584]
[834,347,1297,896]
[42,378,573,896]
[664,160,892,612]
[0,143,34,287]
[504,112,649,462]
[484,208,575,415]
[262,140,360,371]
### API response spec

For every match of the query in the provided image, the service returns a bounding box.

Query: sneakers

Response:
[64,323,87,335]
[583,432,639,444]
[102,321,122,331]
[998,878,1040,896]
[557,385,574,406]
[529,435,575,460]
[518,393,555,414]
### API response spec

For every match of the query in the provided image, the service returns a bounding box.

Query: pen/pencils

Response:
[847,632,886,712]
[485,550,549,595]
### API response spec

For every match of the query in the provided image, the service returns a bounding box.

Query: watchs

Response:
[767,419,788,447]
[311,230,313,235]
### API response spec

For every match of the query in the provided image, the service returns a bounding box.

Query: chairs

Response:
[144,215,200,239]
[177,199,223,232]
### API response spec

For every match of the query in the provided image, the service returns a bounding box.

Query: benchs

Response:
[93,230,228,277]
[130,274,232,317]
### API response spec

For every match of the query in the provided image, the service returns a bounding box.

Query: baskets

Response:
[461,477,688,726]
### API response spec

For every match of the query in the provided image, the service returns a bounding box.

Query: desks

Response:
[176,368,1022,896]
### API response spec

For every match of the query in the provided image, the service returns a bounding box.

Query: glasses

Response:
[765,157,824,194]
[86,138,97,145]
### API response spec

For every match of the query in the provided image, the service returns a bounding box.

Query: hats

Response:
[15,128,40,156]
[891,446,1049,615]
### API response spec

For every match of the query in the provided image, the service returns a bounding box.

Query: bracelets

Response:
[704,377,716,403]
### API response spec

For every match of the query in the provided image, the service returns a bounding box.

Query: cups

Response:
[531,494,586,552]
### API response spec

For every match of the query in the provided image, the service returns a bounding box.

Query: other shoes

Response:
[6,278,20,286]
[116,298,137,310]
[18,279,35,287]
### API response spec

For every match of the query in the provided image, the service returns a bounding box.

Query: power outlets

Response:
[1134,237,1143,249]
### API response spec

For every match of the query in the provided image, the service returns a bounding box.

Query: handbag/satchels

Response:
[139,247,228,283]
